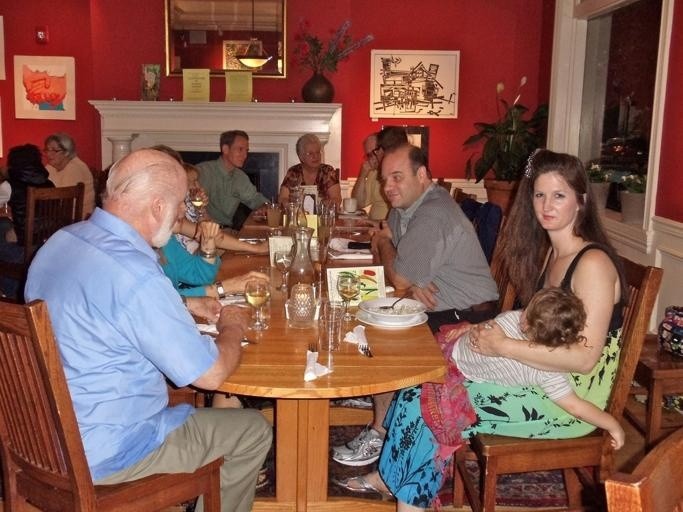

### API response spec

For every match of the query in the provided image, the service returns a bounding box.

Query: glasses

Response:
[44,150,65,157]
[302,149,323,157]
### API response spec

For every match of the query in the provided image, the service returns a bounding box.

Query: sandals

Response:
[331,472,390,495]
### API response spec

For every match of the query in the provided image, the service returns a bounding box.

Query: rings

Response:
[471,339,478,346]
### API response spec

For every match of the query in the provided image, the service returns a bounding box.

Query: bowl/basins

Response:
[358,297,428,321]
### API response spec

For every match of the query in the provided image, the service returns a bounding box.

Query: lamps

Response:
[235,0,273,67]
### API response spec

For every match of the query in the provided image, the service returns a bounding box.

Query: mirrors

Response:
[165,1,288,79]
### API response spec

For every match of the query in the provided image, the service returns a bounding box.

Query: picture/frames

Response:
[223,40,263,74]
[369,49,460,122]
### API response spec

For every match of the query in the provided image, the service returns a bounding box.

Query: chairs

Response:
[604,427,683,512]
[2,182,85,299]
[1,299,225,511]
[437,181,500,265]
[490,217,535,318]
[453,256,663,512]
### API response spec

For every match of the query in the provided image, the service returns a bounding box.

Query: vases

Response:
[587,182,610,212]
[619,191,645,227]
[301,71,333,102]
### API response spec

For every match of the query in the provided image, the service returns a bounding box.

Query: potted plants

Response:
[463,76,549,217]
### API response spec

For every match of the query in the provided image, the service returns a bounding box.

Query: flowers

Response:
[586,160,617,182]
[289,17,374,72]
[618,169,649,193]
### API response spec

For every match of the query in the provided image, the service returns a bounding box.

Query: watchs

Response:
[263,202,269,206]
[215,281,224,298]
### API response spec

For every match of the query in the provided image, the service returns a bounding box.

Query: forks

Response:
[308,342,318,353]
[360,344,373,358]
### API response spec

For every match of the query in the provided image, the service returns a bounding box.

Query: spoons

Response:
[379,290,413,309]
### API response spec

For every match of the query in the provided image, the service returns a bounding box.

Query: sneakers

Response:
[341,395,374,409]
[330,429,381,466]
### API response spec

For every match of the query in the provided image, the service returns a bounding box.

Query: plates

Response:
[355,311,428,330]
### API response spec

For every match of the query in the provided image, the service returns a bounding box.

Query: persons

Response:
[276,134,342,209]
[175,165,208,254]
[331,148,625,512]
[350,134,379,210]
[450,286,626,450]
[151,145,269,256]
[22,148,272,511]
[94,162,271,490]
[193,130,271,235]
[0,143,59,297]
[329,141,501,467]
[369,127,408,221]
[43,132,95,222]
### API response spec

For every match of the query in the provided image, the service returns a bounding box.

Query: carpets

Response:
[333,395,606,512]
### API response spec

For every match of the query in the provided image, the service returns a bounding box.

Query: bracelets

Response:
[180,295,187,307]
[360,172,366,177]
[199,251,217,258]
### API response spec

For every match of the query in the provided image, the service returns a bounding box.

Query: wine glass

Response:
[189,188,204,217]
[244,187,361,330]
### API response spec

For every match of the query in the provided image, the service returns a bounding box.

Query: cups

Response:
[319,315,342,351]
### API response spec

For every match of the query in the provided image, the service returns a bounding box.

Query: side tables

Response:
[624,333,683,457]
